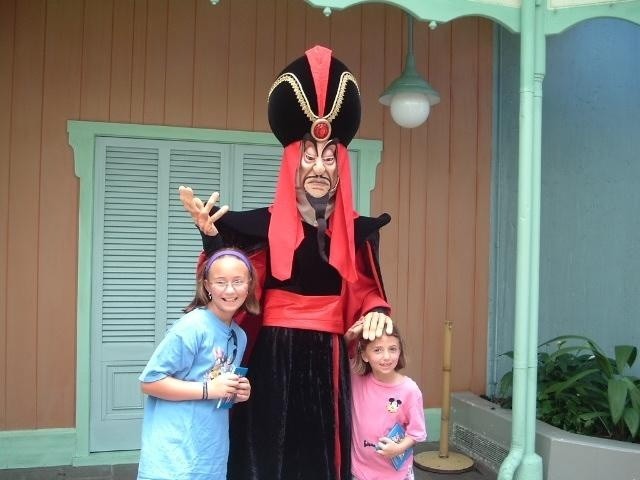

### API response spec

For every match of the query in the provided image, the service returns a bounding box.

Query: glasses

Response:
[208,278,248,288]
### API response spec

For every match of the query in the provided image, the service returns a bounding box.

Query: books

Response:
[217,368,248,409]
[384,424,415,470]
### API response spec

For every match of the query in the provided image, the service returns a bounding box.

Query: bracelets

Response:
[202,381,208,400]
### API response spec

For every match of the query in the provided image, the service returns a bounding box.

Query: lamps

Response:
[375,8,440,130]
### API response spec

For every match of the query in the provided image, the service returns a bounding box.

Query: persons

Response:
[342,317,427,479]
[179,44,393,480]
[134,248,255,480]
